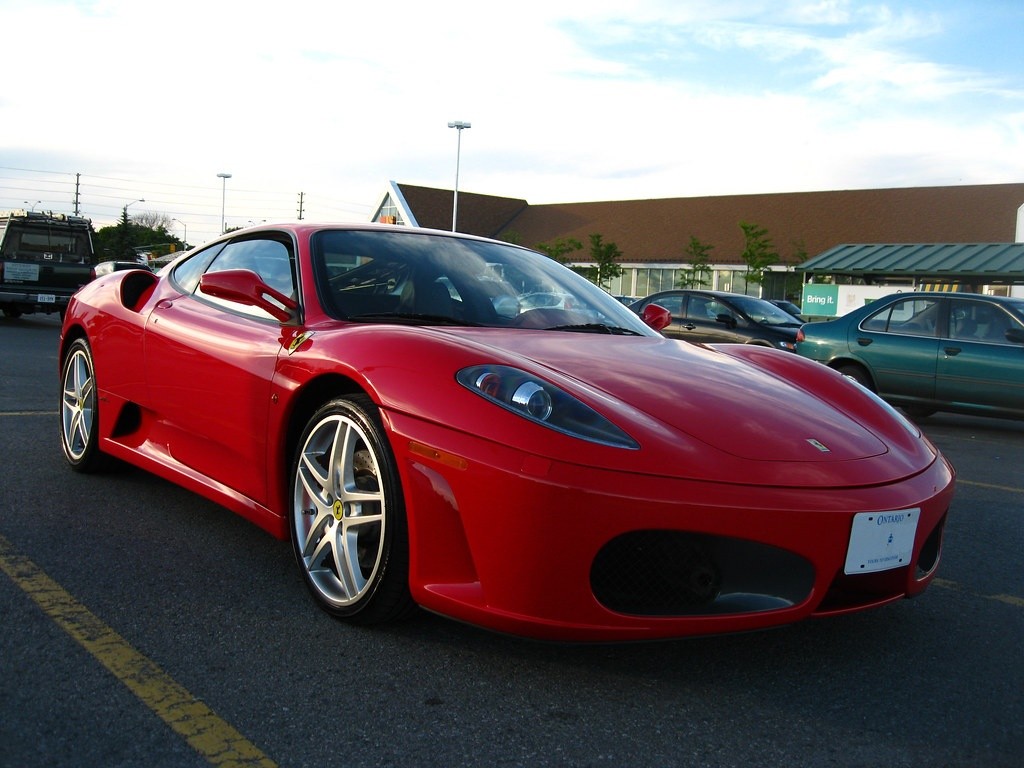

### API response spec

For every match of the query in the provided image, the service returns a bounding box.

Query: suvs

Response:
[391,277,520,317]
[0,213,96,323]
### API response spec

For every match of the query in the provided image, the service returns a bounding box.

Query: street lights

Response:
[172,218,187,251]
[216,173,232,235]
[447,120,471,233]
[24,200,42,213]
[126,199,146,223]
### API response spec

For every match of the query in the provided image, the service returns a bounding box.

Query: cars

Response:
[796,291,1024,422]
[95,261,154,277]
[518,291,584,315]
[599,289,801,354]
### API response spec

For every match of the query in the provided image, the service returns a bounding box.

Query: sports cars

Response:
[55,221,956,640]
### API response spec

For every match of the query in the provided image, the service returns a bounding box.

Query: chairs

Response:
[251,249,1012,344]
